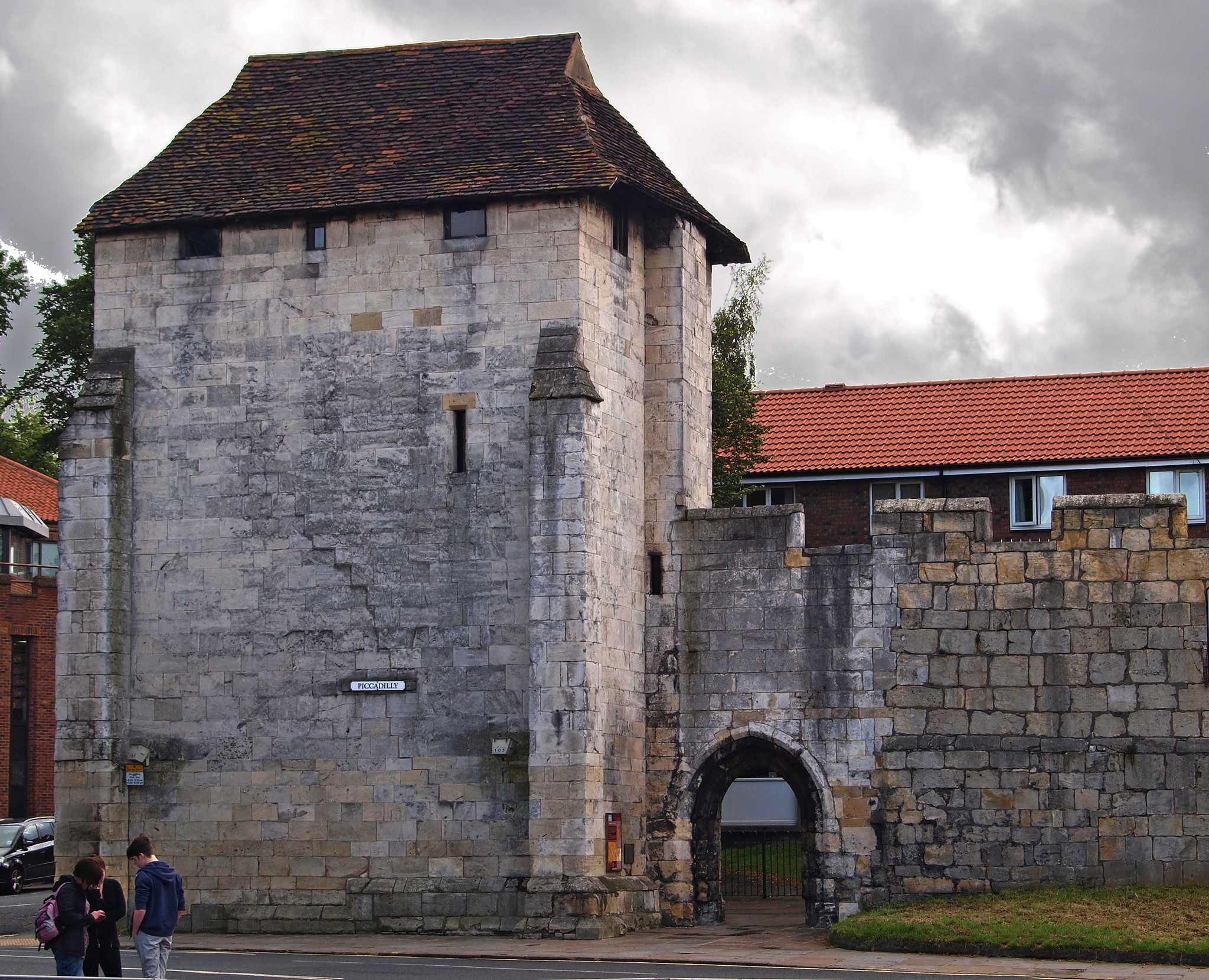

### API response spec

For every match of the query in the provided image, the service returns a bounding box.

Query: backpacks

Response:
[35,882,73,943]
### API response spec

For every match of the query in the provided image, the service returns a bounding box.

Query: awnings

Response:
[1,497,49,538]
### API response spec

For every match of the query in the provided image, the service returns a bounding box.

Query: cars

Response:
[0,816,58,894]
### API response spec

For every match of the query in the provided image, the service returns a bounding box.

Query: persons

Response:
[44,857,107,977]
[126,832,186,980]
[82,854,126,978]
